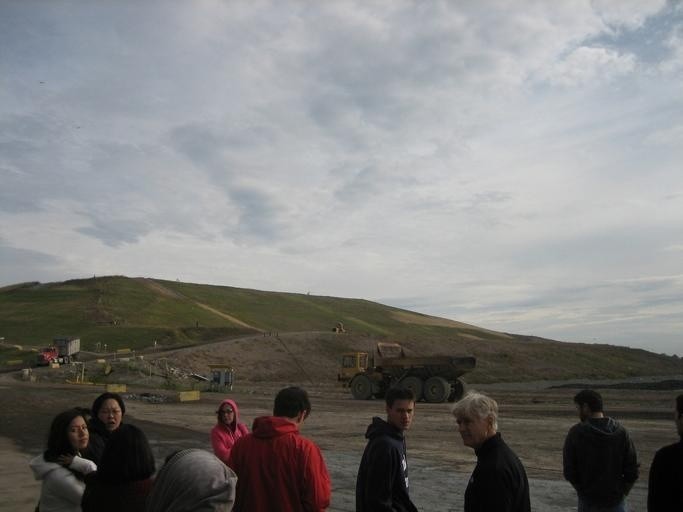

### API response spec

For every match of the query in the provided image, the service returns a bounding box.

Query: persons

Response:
[30,391,155,511]
[647,395,683,512]
[147,399,248,511]
[453,390,530,512]
[356,387,418,512]
[563,390,641,512]
[228,387,328,511]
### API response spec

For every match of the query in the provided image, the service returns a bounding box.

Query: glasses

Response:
[220,408,233,415]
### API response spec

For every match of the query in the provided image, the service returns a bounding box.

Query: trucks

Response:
[36,331,80,365]
[337,342,477,401]
